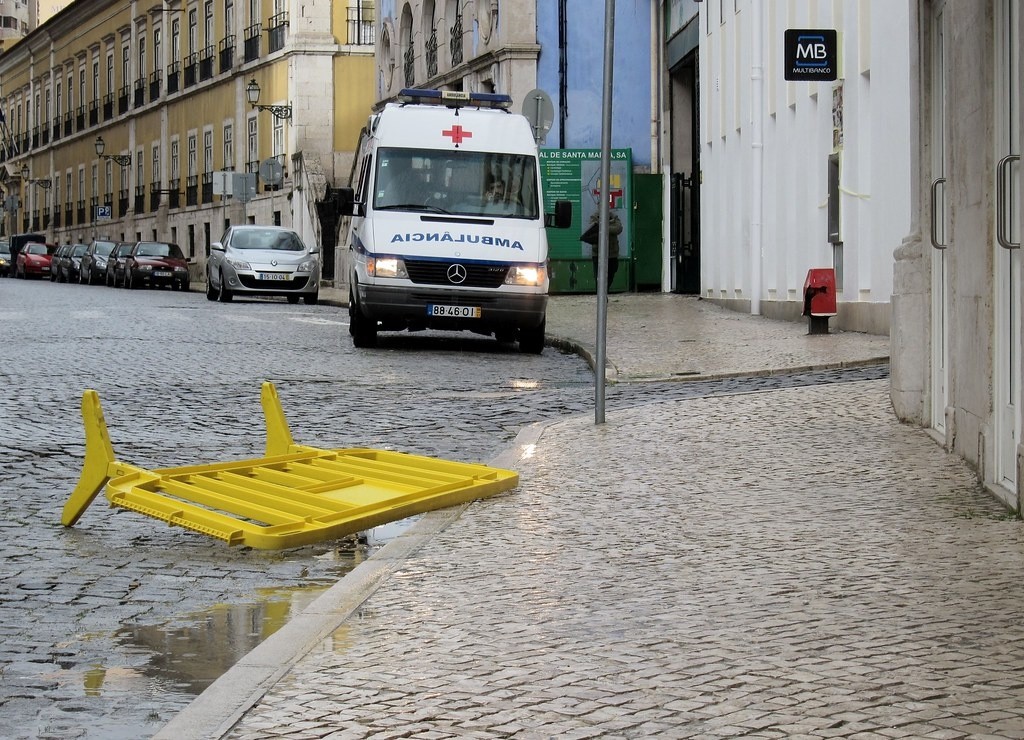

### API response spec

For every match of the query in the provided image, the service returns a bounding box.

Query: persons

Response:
[484,172,521,205]
[589,198,623,302]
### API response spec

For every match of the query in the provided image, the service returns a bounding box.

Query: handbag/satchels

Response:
[580,222,599,245]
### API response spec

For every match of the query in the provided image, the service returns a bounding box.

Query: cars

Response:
[124,240,191,292]
[106,241,136,285]
[79,239,119,283]
[205,224,321,305]
[60,244,88,282]
[50,245,71,280]
[16,241,56,280]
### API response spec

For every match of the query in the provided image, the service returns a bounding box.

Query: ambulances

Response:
[328,88,572,355]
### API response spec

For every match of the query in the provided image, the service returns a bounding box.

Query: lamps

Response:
[94,136,131,166]
[21,164,51,188]
[245,79,292,126]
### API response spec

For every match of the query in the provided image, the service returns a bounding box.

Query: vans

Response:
[0,241,11,275]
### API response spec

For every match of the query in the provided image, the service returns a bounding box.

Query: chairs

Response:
[234,232,290,249]
